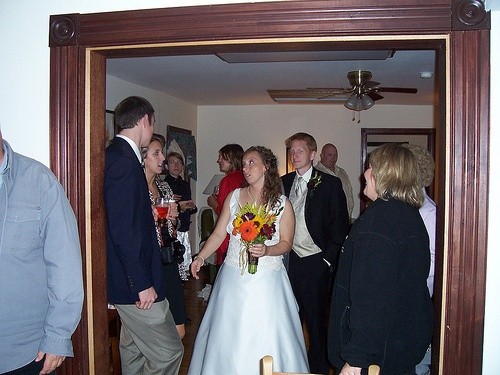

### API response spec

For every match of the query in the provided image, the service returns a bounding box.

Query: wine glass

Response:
[156,197,169,227]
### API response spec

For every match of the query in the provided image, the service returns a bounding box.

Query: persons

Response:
[0,129,85,375]
[327,142,435,375]
[402,143,438,296]
[103,96,185,375]
[140,133,187,340]
[206,144,249,263]
[164,152,198,279]
[280,133,349,375]
[314,143,355,220]
[187,145,310,375]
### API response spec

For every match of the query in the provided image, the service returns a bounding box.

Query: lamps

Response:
[344,93,374,123]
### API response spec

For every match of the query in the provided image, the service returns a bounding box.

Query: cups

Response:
[214,186,219,198]
[165,200,176,220]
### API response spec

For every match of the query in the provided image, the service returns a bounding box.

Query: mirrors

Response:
[361,127,436,211]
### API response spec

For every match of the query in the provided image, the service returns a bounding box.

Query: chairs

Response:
[200,208,217,285]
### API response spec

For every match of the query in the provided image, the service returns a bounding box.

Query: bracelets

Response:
[194,256,204,266]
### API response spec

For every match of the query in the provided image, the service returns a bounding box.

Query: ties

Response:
[297,177,302,198]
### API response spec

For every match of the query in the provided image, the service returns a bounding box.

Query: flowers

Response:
[232,203,276,274]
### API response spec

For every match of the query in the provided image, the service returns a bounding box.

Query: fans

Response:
[307,71,417,101]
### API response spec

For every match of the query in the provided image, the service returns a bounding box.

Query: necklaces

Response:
[247,185,253,200]
